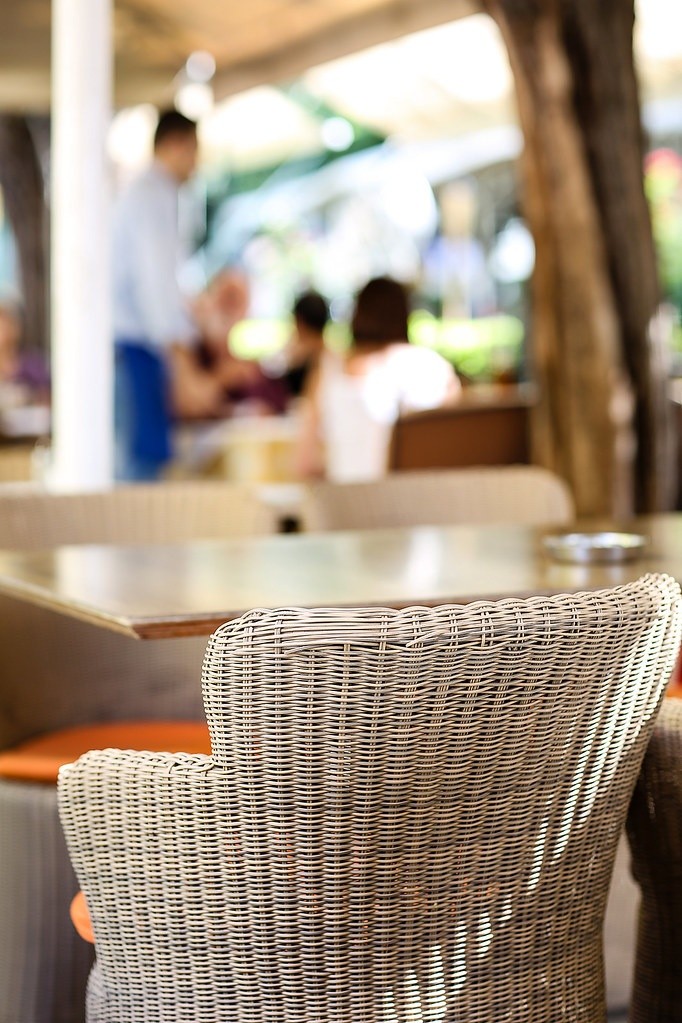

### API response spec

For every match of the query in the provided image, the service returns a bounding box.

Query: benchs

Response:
[0,466,576,1023]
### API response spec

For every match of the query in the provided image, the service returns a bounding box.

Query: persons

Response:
[0,302,49,442]
[110,106,201,485]
[174,258,463,484]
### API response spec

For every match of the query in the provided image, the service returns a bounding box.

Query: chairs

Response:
[58,572,682,1023]
[624,699,682,1023]
[386,404,529,473]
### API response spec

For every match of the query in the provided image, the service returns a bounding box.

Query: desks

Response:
[0,512,681,643]
